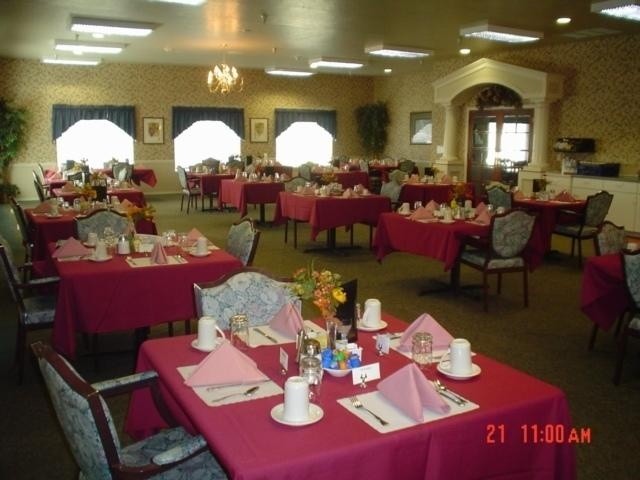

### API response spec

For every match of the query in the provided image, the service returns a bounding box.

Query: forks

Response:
[348,393,389,427]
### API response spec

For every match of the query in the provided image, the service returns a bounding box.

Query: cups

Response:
[411,333,433,371]
[192,237,208,255]
[88,233,97,245]
[439,338,474,375]
[230,315,248,351]
[198,317,225,350]
[403,203,409,213]
[444,210,452,222]
[284,376,309,422]
[299,358,323,401]
[363,299,382,328]
[95,242,106,259]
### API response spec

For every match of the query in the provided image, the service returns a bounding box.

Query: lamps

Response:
[459,24,544,43]
[590,0,640,22]
[264,67,316,77]
[364,43,434,59]
[40,55,102,66]
[52,38,126,55]
[65,15,157,38]
[207,53,244,95]
[309,56,366,70]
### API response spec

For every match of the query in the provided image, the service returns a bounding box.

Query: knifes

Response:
[253,328,277,344]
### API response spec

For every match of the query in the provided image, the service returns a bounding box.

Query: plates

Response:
[357,319,388,332]
[440,219,455,223]
[190,251,212,257]
[399,212,410,215]
[191,337,231,352]
[83,242,97,247]
[271,402,325,427]
[89,255,112,262]
[48,215,63,217]
[437,361,480,380]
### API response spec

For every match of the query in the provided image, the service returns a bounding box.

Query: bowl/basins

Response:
[324,368,352,378]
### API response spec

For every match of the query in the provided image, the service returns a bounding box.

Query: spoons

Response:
[213,386,259,403]
[437,378,469,406]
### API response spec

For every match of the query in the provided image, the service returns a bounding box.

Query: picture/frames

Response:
[410,110,432,146]
[141,116,163,145]
[250,118,269,144]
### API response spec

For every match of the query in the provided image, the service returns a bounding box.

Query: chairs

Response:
[282,176,320,251]
[177,165,213,215]
[112,163,133,179]
[359,159,381,193]
[226,161,244,171]
[381,155,394,162]
[488,185,513,210]
[484,181,510,192]
[264,167,277,177]
[202,157,219,173]
[33,181,63,208]
[10,198,33,292]
[38,163,50,196]
[91,176,107,202]
[169,216,262,334]
[587,220,628,354]
[29,340,228,480]
[0,243,90,386]
[399,160,415,175]
[455,208,539,314]
[32,169,55,200]
[351,178,402,250]
[612,248,640,385]
[69,172,92,182]
[552,188,613,270]
[194,266,309,331]
[300,164,311,180]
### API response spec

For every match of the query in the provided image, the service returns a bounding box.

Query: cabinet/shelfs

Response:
[602,179,639,237]
[573,177,601,202]
[543,172,570,198]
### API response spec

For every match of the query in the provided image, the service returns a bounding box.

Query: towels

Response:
[50,236,90,259]
[376,362,451,425]
[342,188,359,199]
[425,199,439,211]
[151,242,170,266]
[475,202,487,212]
[267,300,308,340]
[32,200,56,213]
[183,339,270,387]
[118,197,139,212]
[409,206,433,221]
[397,313,454,353]
[475,209,492,225]
[183,227,213,247]
